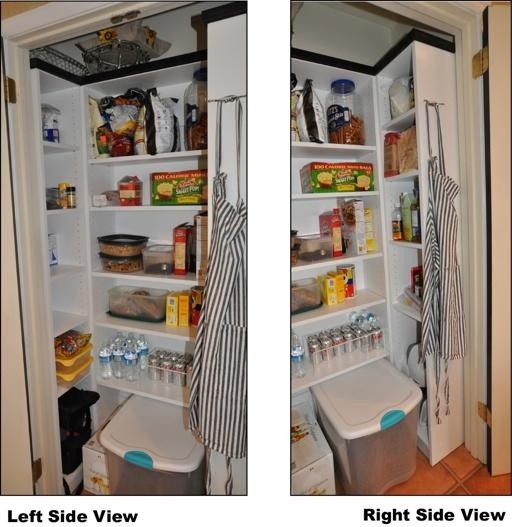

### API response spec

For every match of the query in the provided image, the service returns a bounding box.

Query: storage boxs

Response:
[311,358,424,496]
[291,402,337,495]
[81,404,121,496]
[96,394,209,495]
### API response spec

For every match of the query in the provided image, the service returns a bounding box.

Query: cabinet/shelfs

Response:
[0,1,246,496]
[290,1,512,496]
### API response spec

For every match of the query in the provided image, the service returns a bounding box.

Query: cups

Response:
[337,264,356,297]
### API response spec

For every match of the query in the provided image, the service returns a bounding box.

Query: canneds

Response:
[149,350,193,388]
[308,322,385,367]
[58,183,76,209]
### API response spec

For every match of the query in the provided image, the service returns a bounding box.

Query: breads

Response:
[316,170,372,191]
[158,182,208,203]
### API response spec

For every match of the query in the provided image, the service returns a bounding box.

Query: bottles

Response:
[181,66,209,151]
[52,182,76,208]
[323,76,366,145]
[97,331,150,382]
[291,330,307,379]
[390,190,420,240]
[346,307,379,331]
[334,208,346,254]
[41,101,63,145]
